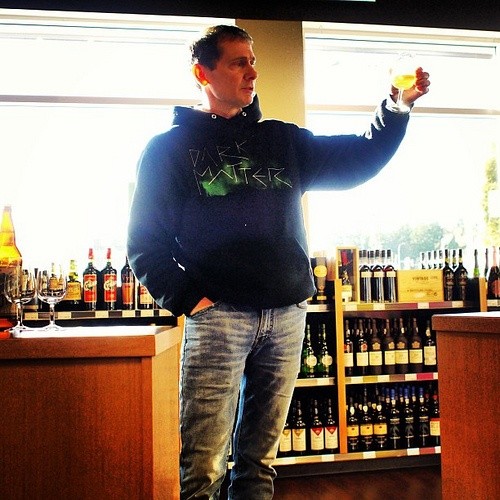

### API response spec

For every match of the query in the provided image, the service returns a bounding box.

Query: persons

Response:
[128,24,429,499]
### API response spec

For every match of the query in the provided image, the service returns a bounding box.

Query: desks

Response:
[0,325,183,500]
[431,310,500,500]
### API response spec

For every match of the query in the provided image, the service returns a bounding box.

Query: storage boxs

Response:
[330,246,361,304]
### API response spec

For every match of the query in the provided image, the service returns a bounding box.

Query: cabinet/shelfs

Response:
[0,271,500,479]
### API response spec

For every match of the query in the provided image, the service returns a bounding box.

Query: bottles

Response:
[340,264,352,302]
[359,249,399,303]
[419,246,500,302]
[138,282,154,310]
[23,259,81,311]
[345,316,440,453]
[120,255,135,310]
[277,322,340,459]
[0,202,23,315]
[80,248,101,310]
[102,247,117,310]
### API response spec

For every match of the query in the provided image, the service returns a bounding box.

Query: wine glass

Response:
[36,262,66,331]
[3,256,36,335]
